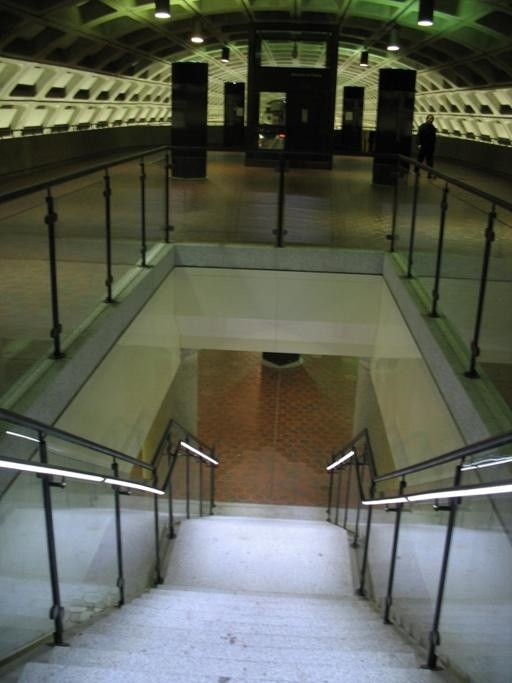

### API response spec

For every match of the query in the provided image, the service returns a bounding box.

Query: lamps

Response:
[153,1,435,68]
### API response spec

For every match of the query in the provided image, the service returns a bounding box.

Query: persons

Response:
[413,114,436,179]
